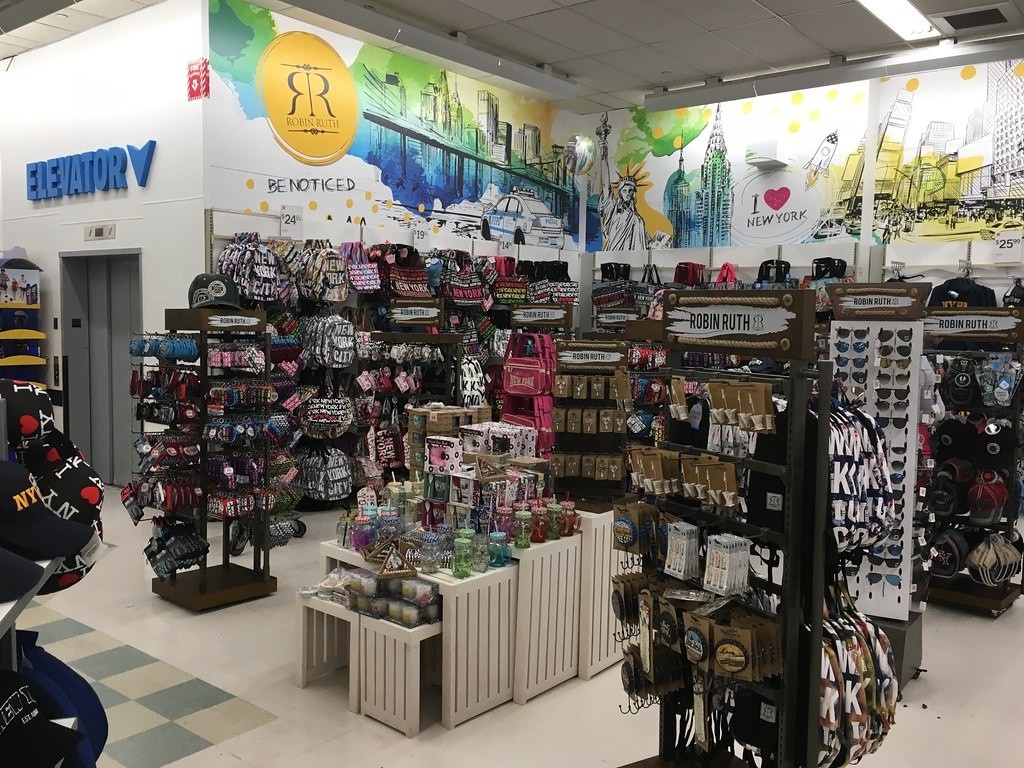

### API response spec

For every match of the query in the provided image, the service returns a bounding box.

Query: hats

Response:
[926,357,1024,587]
[1,549,44,603]
[0,670,86,768]
[0,460,95,561]
[188,274,243,311]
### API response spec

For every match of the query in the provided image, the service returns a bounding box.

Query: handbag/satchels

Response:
[501,334,555,458]
[591,258,856,319]
[813,383,899,767]
[298,307,353,502]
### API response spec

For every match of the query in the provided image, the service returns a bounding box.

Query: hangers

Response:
[892,269,925,281]
[961,269,969,282]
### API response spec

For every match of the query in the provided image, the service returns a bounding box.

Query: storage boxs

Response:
[406,405,553,531]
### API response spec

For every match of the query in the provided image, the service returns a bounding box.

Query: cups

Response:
[451,539,473,579]
[495,507,514,539]
[514,511,533,549]
[489,532,512,567]
[512,502,530,513]
[546,504,563,540]
[531,506,549,543]
[472,533,491,573]
[541,496,557,508]
[337,477,426,552]
[559,501,582,536]
[421,533,442,574]
[526,497,542,509]
[459,528,475,540]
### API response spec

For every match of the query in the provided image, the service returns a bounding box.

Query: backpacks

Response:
[218,232,580,307]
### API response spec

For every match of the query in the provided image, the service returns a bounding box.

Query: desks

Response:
[283,509,644,740]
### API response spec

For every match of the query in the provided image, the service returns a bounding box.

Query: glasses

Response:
[833,327,913,587]
